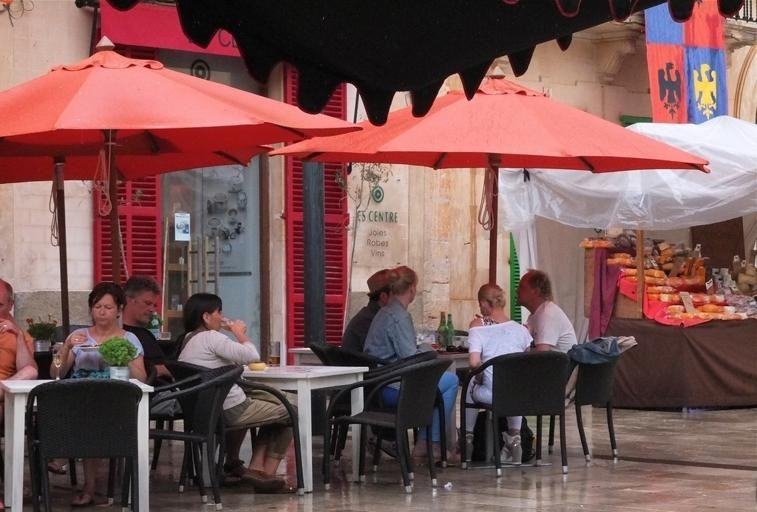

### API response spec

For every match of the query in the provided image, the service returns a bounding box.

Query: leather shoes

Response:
[370,435,398,458]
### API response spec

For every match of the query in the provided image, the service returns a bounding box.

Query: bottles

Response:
[149,304,165,332]
[438,311,449,349]
[447,313,455,335]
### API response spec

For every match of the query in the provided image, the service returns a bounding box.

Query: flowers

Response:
[26,312,57,342]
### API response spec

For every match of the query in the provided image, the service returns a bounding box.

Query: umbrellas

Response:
[0,35,363,348]
[266,67,710,283]
[0,133,280,341]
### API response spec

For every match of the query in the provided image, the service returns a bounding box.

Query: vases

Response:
[35,340,51,353]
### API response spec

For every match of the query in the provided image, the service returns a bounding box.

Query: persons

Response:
[0,278,39,509]
[340,270,392,407]
[170,293,298,493]
[50,283,146,507]
[363,267,458,468]
[457,284,533,465]
[516,270,579,406]
[122,275,184,418]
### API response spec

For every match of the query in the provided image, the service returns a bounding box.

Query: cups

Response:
[51,344,64,382]
[431,332,441,351]
[268,341,280,368]
[220,317,233,330]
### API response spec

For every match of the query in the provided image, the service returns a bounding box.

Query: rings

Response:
[75,337,78,341]
[4,324,7,328]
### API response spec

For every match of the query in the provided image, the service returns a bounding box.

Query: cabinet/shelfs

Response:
[585,230,756,408]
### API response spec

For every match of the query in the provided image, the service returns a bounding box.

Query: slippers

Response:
[69,491,97,505]
[413,446,452,467]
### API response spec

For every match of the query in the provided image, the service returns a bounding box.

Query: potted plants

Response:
[101,339,136,381]
[145,311,164,340]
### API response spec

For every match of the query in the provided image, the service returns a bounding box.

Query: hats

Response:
[367,269,391,296]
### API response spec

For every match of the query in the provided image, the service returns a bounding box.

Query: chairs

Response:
[149,413,185,470]
[349,353,446,471]
[322,350,437,479]
[164,360,304,496]
[27,379,142,512]
[37,365,156,511]
[309,341,419,458]
[121,363,244,511]
[534,334,636,461]
[324,357,452,493]
[460,352,568,475]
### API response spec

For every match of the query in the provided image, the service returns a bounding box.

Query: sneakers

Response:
[241,467,285,490]
[222,467,251,486]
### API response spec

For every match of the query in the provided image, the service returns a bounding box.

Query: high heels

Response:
[490,431,523,463]
[455,433,474,462]
